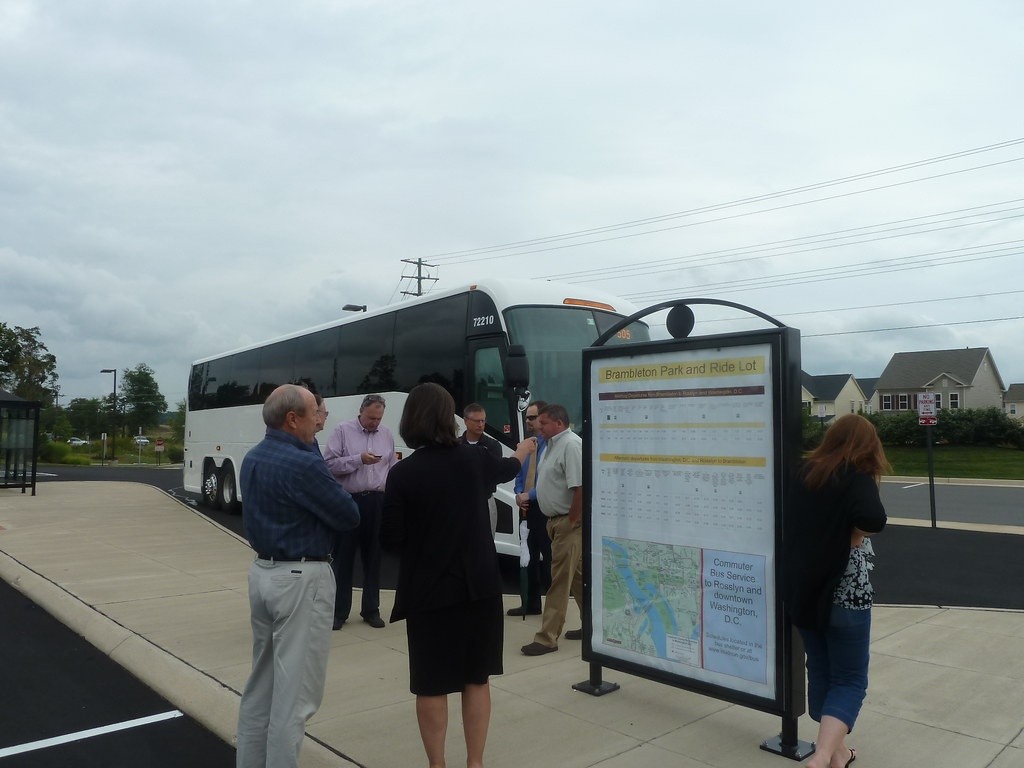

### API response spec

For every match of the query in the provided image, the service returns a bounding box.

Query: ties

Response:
[523,440,538,493]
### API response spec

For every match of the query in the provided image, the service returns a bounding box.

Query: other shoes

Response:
[507,607,542,615]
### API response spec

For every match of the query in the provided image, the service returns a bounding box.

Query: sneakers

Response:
[333,618,345,629]
[364,616,385,627]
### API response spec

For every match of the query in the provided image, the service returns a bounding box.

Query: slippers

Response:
[831,749,855,768]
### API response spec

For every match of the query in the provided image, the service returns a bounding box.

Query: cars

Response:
[67,437,88,447]
[133,435,149,445]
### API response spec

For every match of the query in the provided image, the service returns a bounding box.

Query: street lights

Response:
[99,368,117,461]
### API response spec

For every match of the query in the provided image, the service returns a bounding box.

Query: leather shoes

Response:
[565,629,581,639]
[521,642,558,655]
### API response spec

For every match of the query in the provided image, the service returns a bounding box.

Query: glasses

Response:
[465,416,488,425]
[319,411,328,417]
[525,415,539,421]
[363,396,385,403]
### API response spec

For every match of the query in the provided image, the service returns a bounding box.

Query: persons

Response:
[311,393,328,460]
[521,404,582,656]
[457,403,503,540]
[782,414,895,768]
[380,382,537,768]
[505,400,553,616]
[323,395,398,631]
[237,384,362,768]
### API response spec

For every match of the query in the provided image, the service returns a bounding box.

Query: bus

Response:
[181,275,655,566]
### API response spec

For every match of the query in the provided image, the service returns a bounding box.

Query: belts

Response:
[261,551,327,561]
[548,514,567,518]
[351,491,380,499]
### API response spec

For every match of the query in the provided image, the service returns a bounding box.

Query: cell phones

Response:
[374,455,382,459]
